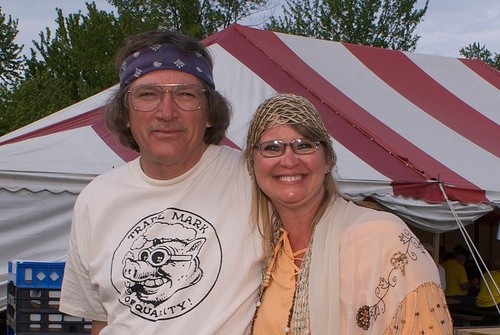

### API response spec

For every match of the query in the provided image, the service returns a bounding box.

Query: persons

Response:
[441,247,480,307]
[476,256,500,314]
[59,30,276,335]
[246,93,454,335]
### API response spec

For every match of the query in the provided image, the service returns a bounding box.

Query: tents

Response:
[0,23,500,316]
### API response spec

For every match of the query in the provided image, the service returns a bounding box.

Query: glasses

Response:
[254,138,322,157]
[126,84,207,112]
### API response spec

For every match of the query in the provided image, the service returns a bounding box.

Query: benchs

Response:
[453,314,482,327]
